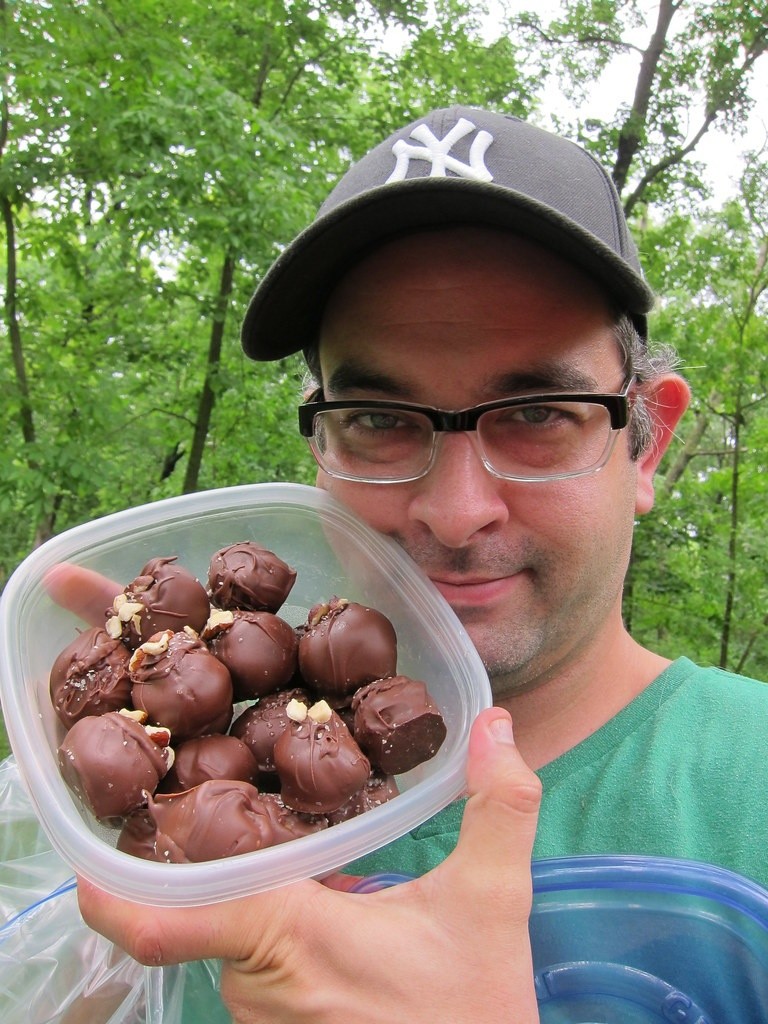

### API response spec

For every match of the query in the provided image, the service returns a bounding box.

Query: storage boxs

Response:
[0,483,496,906]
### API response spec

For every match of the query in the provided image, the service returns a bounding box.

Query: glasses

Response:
[298,373,636,485]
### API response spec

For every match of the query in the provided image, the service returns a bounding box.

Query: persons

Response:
[0,107,767,1023]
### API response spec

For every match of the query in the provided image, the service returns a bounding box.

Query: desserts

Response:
[50,539,448,864]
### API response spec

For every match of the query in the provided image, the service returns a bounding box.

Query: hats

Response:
[240,106,654,363]
